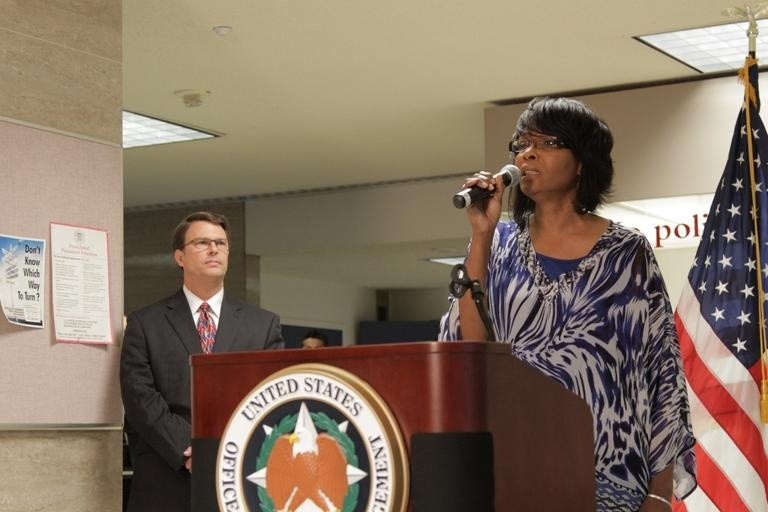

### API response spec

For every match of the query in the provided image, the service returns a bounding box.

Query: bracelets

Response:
[646,493,673,510]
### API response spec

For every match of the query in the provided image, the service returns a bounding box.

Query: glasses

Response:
[509,138,564,154]
[180,238,232,251]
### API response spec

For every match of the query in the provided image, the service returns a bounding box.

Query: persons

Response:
[120,211,286,511]
[302,332,328,349]
[436,97,698,512]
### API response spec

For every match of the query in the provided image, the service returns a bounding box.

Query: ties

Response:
[197,302,217,353]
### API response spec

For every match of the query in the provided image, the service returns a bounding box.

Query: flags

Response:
[670,54,768,512]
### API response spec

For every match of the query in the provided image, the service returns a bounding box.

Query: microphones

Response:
[453,164,522,209]
